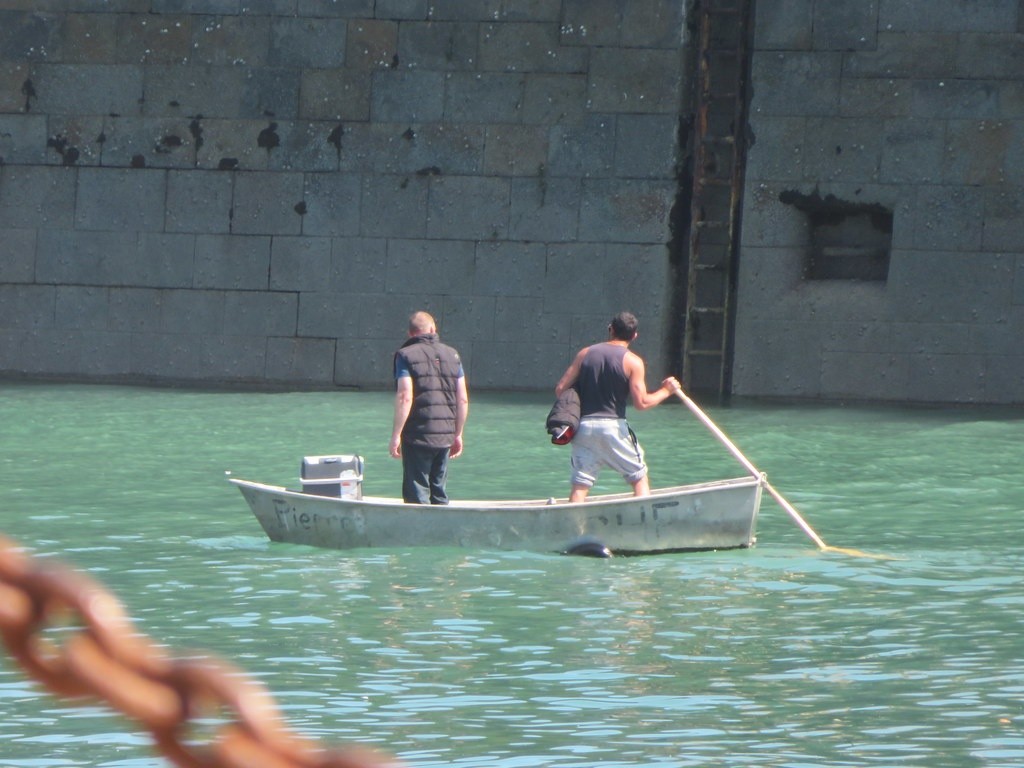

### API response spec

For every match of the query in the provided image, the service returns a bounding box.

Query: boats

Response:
[229,454,768,559]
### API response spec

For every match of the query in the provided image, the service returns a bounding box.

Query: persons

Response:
[555,313,681,502]
[388,311,468,504]
